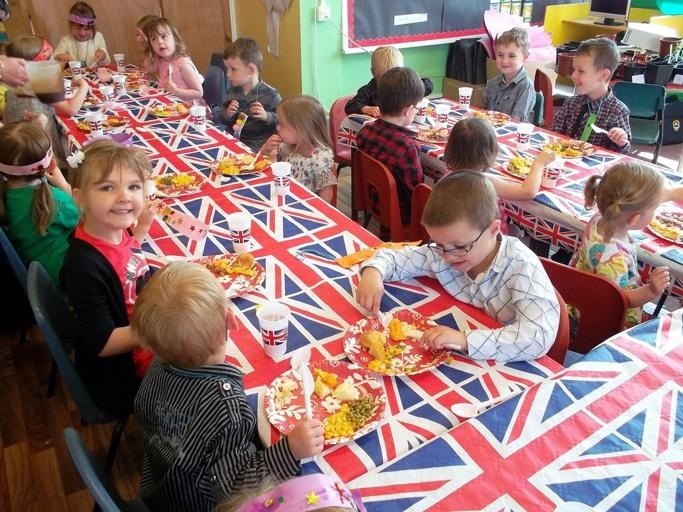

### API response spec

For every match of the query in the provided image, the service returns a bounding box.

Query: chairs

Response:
[534,67,556,130]
[530,91,545,126]
[28,262,136,492]
[536,256,627,356]
[0,229,58,395]
[546,288,570,365]
[611,81,662,164]
[203,67,339,205]
[328,96,356,205]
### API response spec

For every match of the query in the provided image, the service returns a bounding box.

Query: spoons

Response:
[451,389,523,419]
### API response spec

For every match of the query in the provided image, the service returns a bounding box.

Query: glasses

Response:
[428,216,499,257]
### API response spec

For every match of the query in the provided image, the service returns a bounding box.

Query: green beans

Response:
[345,393,375,426]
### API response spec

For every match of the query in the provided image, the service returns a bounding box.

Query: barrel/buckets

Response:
[558,52,576,77]
[659,36,682,58]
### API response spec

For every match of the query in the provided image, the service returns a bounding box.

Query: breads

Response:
[507,158,533,176]
[545,140,582,158]
[649,219,679,240]
[208,253,259,278]
[315,368,360,403]
[360,317,410,359]
[473,110,493,126]
[419,127,450,144]
[154,171,199,193]
[218,153,271,176]
[151,102,186,119]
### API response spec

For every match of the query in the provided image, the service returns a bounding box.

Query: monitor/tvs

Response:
[589,0,631,26]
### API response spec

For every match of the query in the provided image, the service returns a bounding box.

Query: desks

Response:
[126,178,567,490]
[559,15,632,31]
[340,96,683,309]
[0,64,272,219]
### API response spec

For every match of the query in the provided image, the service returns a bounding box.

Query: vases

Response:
[557,37,683,84]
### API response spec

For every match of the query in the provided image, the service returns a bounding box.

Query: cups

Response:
[146,180,156,204]
[622,60,673,88]
[256,302,289,357]
[270,161,292,196]
[557,52,576,76]
[415,87,596,191]
[658,37,681,58]
[24,60,63,103]
[61,53,207,148]
[227,211,253,253]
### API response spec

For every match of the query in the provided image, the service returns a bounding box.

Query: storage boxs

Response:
[443,57,557,109]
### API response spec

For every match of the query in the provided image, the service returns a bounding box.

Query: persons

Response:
[147,17,213,119]
[479,28,537,124]
[355,169,560,360]
[52,2,111,66]
[344,46,433,116]
[134,14,160,75]
[112,261,323,511]
[259,95,338,204]
[356,66,424,242]
[210,37,282,153]
[2,1,85,276]
[570,161,671,329]
[43,139,156,437]
[551,38,632,154]
[446,117,556,235]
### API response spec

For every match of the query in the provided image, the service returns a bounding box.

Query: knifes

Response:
[590,123,627,143]
[298,363,314,419]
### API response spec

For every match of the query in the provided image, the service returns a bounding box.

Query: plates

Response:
[647,206,683,244]
[342,307,448,377]
[156,171,207,198]
[264,358,389,445]
[191,255,264,298]
[211,153,273,175]
